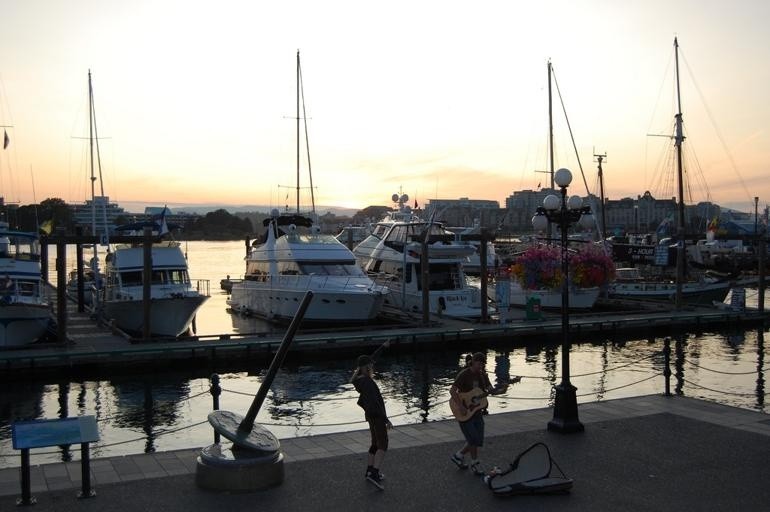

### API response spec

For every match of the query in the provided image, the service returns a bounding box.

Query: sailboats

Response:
[338,35,770,315]
[86,66,212,341]
[225,49,395,328]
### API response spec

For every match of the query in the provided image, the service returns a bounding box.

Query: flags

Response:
[705,214,721,233]
[655,217,673,235]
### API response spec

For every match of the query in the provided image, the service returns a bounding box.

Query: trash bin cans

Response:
[526,295,541,320]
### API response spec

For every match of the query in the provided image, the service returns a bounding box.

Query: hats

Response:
[472,352,487,364]
[358,355,376,367]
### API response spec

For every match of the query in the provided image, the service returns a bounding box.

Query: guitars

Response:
[449,376,521,421]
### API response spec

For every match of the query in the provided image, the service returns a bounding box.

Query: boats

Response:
[0,164,56,350]
[67,255,105,305]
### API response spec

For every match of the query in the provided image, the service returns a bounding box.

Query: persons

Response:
[351,336,391,492]
[448,350,509,476]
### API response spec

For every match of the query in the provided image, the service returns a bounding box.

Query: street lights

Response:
[532,167,596,439]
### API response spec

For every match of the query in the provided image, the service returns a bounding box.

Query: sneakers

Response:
[366,471,385,490]
[470,462,484,475]
[451,454,469,469]
